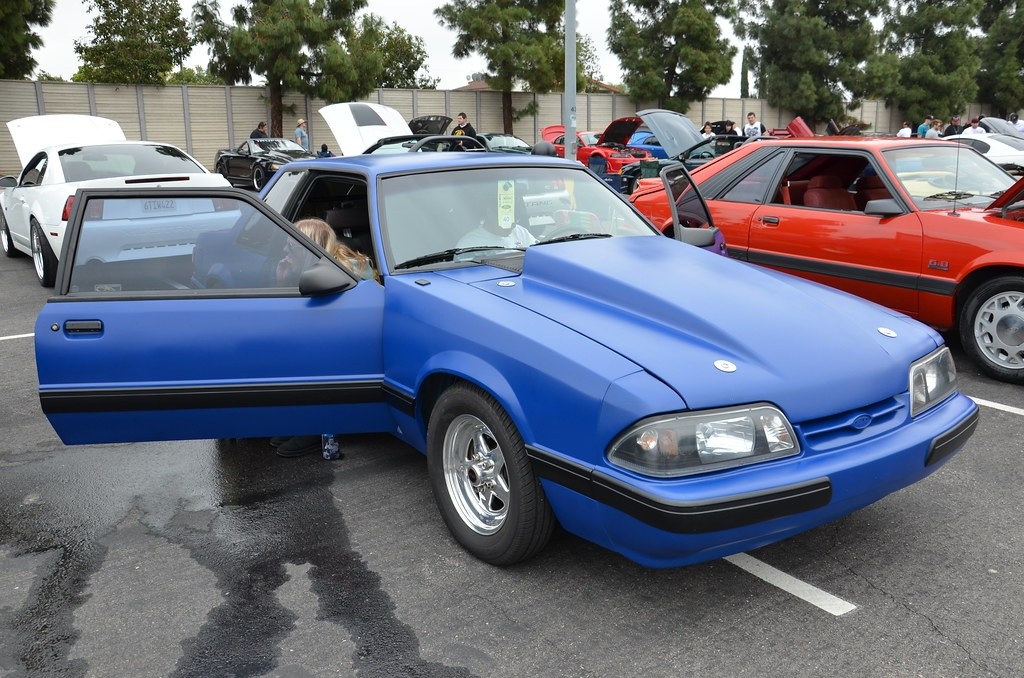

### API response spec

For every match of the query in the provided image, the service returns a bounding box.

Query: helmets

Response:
[1010,113,1018,124]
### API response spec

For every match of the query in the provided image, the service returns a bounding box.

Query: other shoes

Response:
[270,435,292,447]
[276,436,322,457]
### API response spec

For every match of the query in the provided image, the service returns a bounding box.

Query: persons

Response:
[718,120,743,140]
[270,218,382,457]
[895,121,912,138]
[250,121,271,151]
[741,112,769,142]
[450,113,477,152]
[1008,112,1024,132]
[456,186,541,254]
[294,119,308,151]
[319,144,330,158]
[701,121,716,146]
[917,115,990,141]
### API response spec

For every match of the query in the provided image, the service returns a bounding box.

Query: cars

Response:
[543,109,862,213]
[0,114,243,289]
[317,102,534,157]
[626,136,1024,386]
[213,138,318,193]
[945,117,1024,183]
[34,152,980,568]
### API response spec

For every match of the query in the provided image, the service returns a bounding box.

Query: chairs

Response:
[328,201,376,265]
[803,176,858,211]
[857,174,894,208]
[64,160,95,182]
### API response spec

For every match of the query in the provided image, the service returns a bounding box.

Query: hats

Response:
[971,118,978,123]
[979,114,985,120]
[925,115,934,119]
[296,118,307,127]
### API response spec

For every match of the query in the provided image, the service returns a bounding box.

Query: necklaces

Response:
[953,127,960,134]
